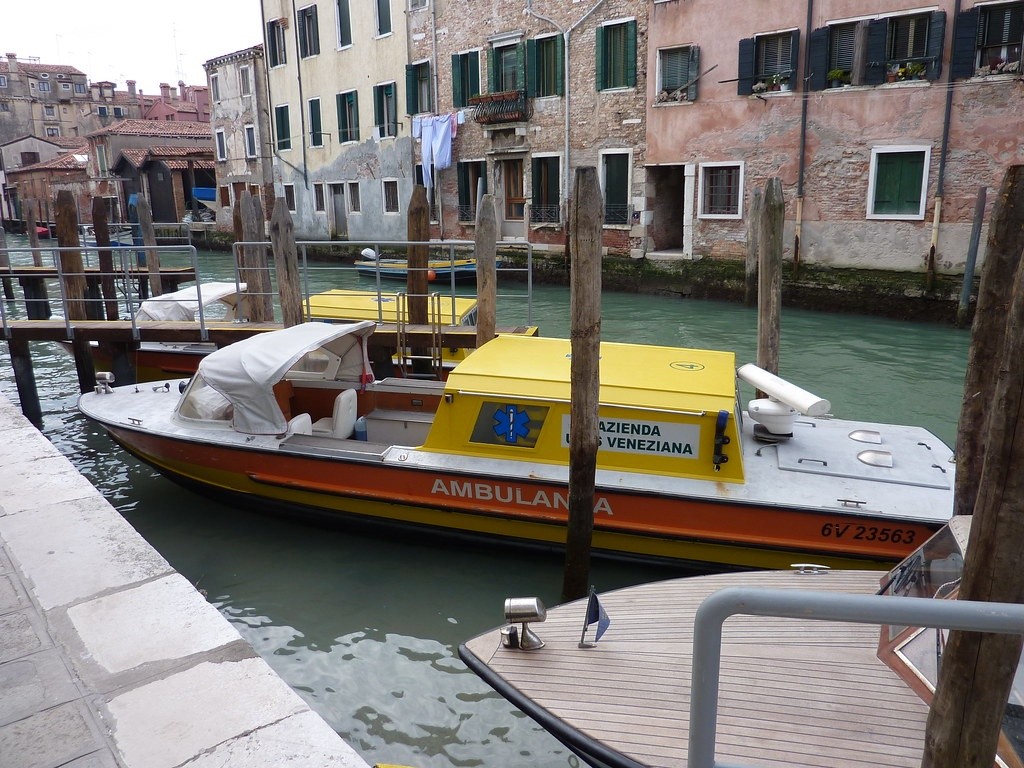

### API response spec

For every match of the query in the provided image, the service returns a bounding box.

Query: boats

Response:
[79,321,958,578]
[78,226,133,250]
[52,282,539,368]
[354,249,501,286]
[457,517,1024,768]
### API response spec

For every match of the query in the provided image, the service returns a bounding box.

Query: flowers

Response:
[772,72,790,85]
[975,60,1019,78]
[896,67,910,81]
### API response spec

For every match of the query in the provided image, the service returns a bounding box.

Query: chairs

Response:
[312,389,358,439]
[286,413,312,435]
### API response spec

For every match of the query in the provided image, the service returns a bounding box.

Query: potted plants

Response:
[752,77,780,93]
[841,70,851,87]
[886,64,896,83]
[826,69,844,87]
[909,61,926,79]
[468,89,518,104]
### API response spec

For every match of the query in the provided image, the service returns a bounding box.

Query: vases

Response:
[897,72,912,80]
[779,83,789,91]
